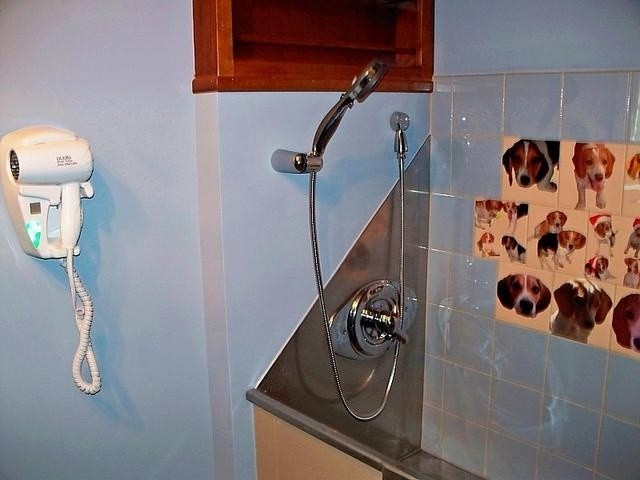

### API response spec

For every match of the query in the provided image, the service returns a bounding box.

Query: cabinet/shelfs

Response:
[192,0,434,94]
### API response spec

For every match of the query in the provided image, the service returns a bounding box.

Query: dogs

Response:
[474,137,640,355]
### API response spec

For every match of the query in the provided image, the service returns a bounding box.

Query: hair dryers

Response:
[10,140,94,249]
